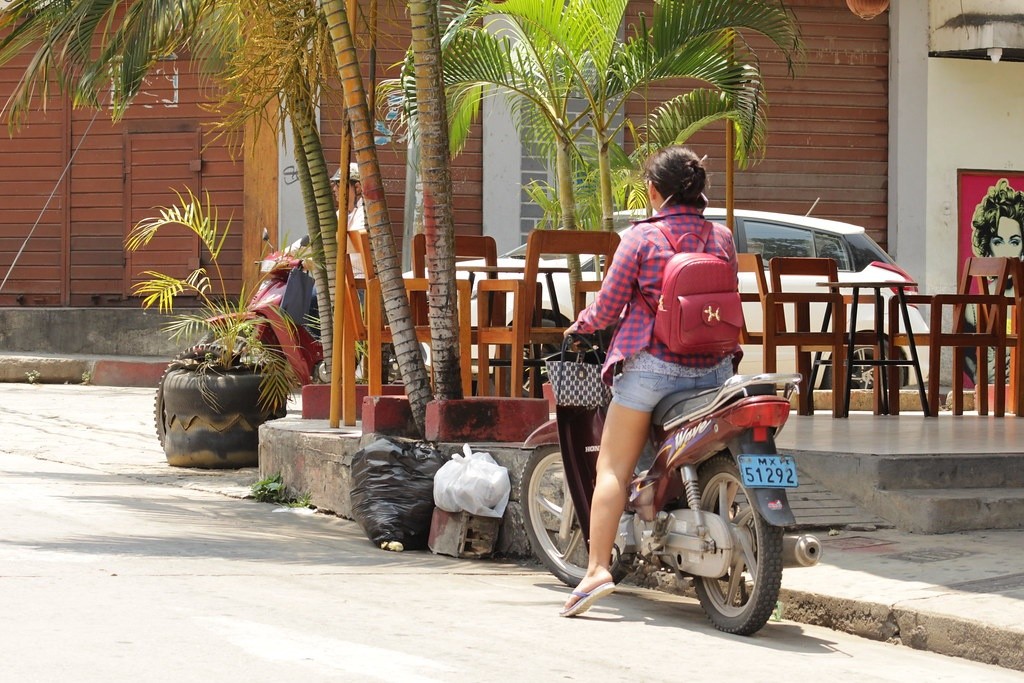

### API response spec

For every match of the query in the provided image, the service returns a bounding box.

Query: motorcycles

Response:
[517,321,824,636]
[191,226,400,386]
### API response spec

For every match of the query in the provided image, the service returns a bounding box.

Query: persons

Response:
[959,178,1024,389]
[559,149,744,618]
[278,162,367,383]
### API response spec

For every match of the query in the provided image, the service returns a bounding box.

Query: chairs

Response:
[476,229,623,397]
[333,229,472,427]
[736,252,845,420]
[975,255,1024,418]
[409,234,508,396]
[887,257,1012,418]
[769,255,885,415]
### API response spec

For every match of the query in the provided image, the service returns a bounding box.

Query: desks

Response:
[806,282,930,417]
[455,266,570,399]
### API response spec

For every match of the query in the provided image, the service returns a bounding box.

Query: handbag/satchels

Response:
[280,261,316,325]
[432,443,511,519]
[545,328,613,407]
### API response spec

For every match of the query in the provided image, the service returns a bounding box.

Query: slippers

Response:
[560,582,615,617]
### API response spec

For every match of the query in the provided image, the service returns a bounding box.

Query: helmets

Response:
[330,163,360,181]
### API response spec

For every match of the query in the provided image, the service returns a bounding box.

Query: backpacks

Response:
[633,218,744,354]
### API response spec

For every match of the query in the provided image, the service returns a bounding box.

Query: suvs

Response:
[398,206,938,401]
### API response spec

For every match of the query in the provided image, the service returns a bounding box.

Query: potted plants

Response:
[122,181,306,471]
